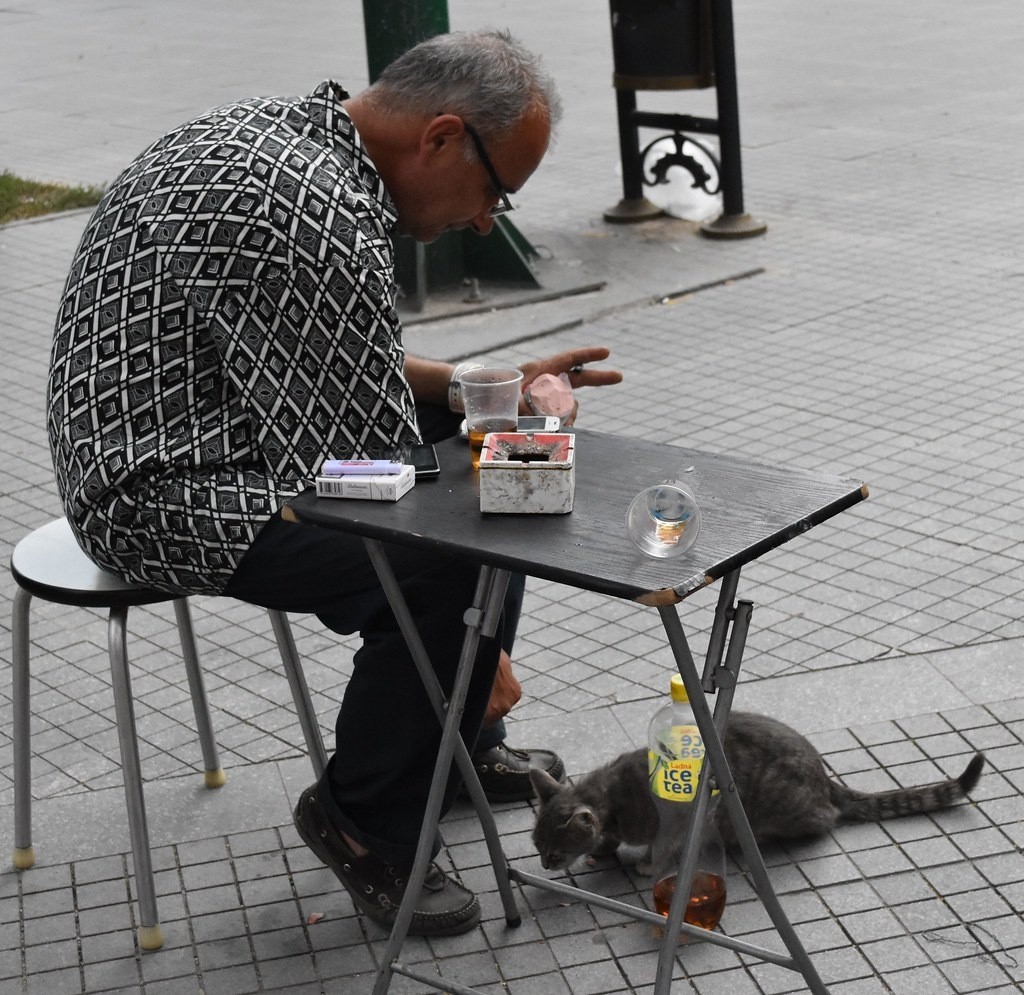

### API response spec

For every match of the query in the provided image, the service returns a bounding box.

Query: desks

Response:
[282,422,869,995]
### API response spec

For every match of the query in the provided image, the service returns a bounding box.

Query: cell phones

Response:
[379,443,441,480]
[456,415,560,441]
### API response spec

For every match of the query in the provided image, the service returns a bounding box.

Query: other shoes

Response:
[479,742,568,801]
[290,783,483,938]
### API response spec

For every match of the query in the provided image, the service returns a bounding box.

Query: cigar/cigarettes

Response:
[573,364,583,374]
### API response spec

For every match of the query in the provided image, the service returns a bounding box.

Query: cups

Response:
[624,480,701,560]
[457,366,524,469]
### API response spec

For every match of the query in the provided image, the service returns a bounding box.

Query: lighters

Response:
[321,460,402,475]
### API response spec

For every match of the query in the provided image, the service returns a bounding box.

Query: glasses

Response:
[434,111,515,217]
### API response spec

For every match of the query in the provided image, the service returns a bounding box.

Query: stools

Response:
[10,515,329,951]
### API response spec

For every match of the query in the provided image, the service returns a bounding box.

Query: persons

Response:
[45,26,624,937]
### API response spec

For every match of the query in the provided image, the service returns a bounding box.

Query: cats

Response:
[525,708,987,878]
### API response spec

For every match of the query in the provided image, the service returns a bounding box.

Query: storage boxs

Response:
[315,464,415,501]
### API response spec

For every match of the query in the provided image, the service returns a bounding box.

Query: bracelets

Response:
[448,362,484,413]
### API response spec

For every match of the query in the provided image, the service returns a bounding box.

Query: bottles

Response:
[648,672,727,932]
